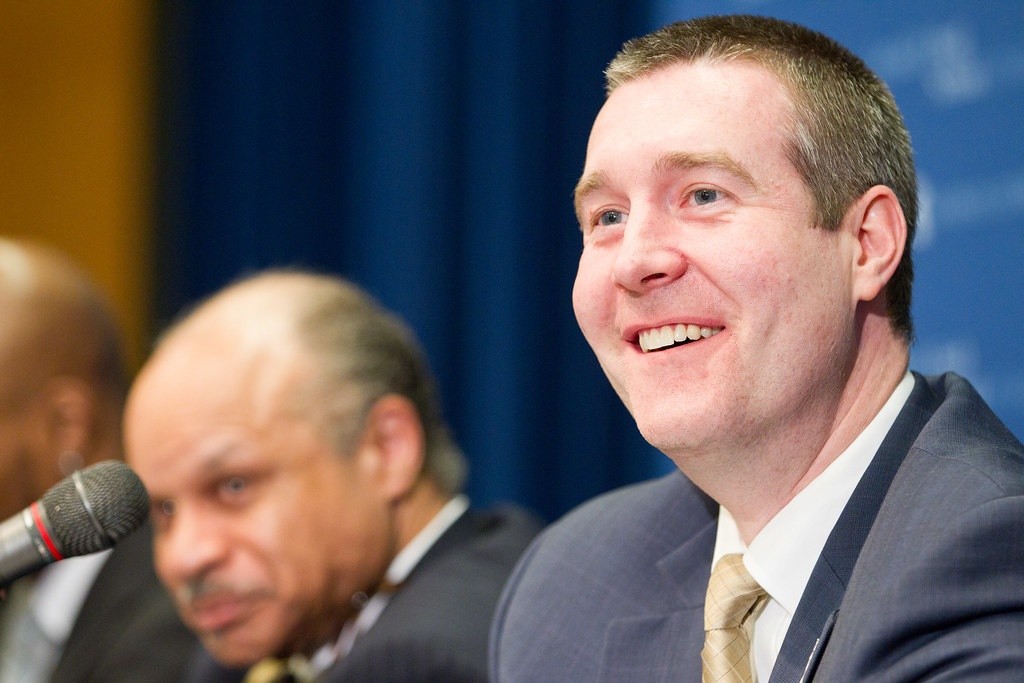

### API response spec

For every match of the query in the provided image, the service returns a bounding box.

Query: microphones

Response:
[0,459,152,588]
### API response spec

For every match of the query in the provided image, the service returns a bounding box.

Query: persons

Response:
[1,239,253,683]
[489,15,1022,683]
[121,267,541,680]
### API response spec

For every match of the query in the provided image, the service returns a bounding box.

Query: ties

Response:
[700,553,771,683]
